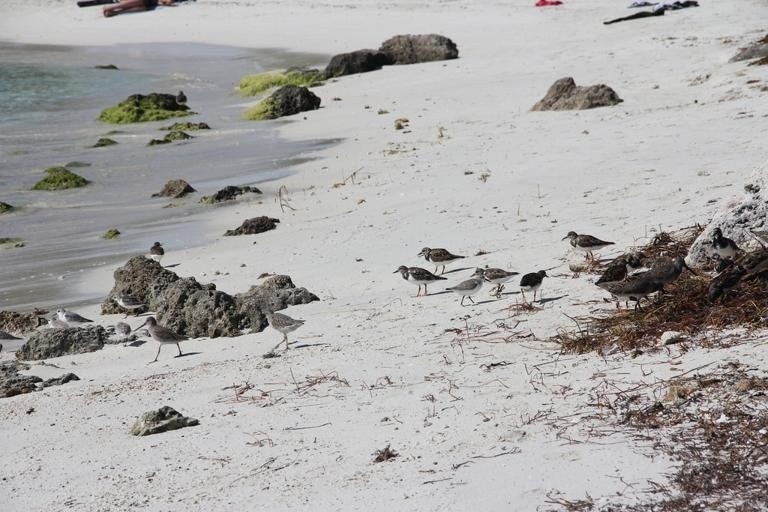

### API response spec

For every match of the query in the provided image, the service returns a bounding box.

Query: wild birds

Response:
[519,269,549,304]
[134,316,191,366]
[470,267,519,296]
[175,90,187,104]
[591,282,617,293]
[150,242,164,265]
[392,265,449,298]
[110,295,150,320]
[260,307,306,350]
[560,231,616,260]
[416,247,468,276]
[46,307,94,330]
[446,274,492,308]
[594,250,699,316]
[710,227,746,262]
